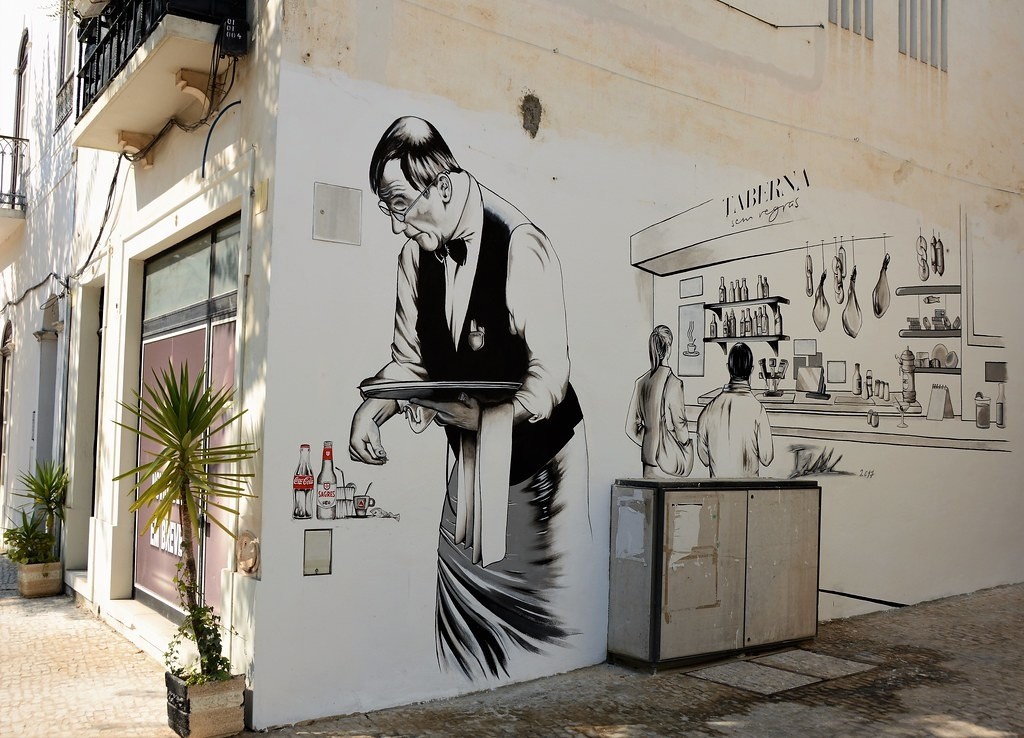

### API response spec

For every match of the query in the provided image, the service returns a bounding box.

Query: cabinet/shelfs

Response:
[608,476,822,671]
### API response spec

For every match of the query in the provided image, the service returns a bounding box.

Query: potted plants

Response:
[0,458,70,600]
[112,356,259,738]
